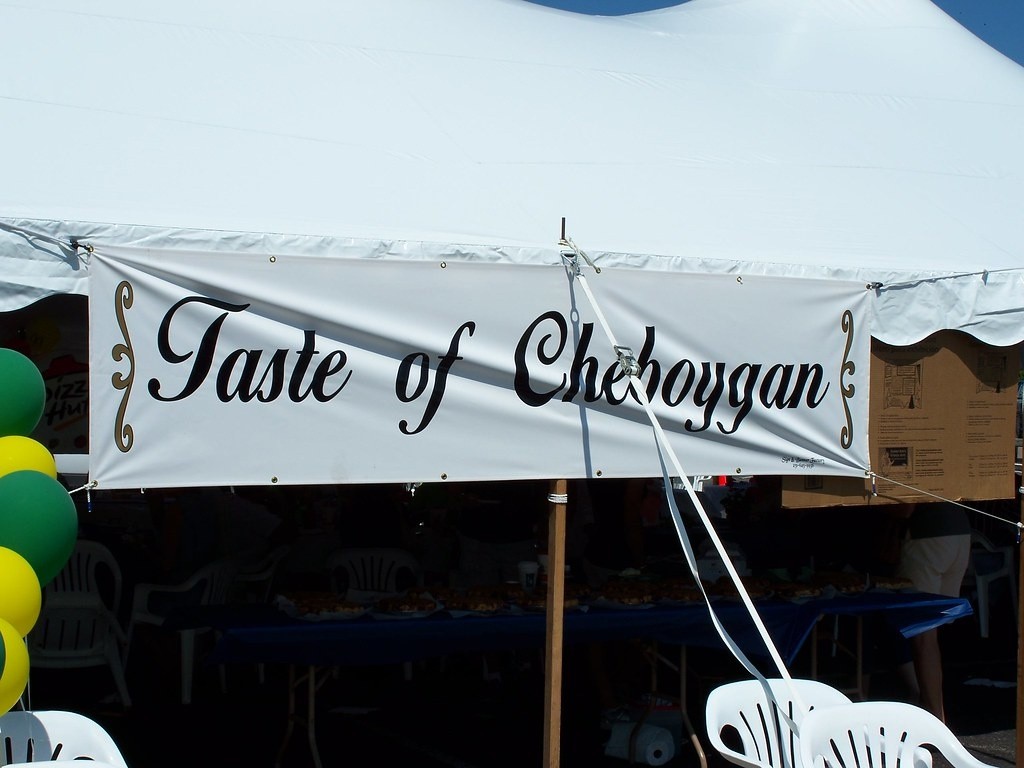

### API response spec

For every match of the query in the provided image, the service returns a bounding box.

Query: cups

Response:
[518,561,540,588]
[504,581,520,602]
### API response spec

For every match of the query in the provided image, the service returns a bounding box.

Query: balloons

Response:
[0,347,78,720]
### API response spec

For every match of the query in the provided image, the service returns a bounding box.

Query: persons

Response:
[901,502,971,723]
[339,485,408,550]
[177,486,298,569]
[886,614,920,704]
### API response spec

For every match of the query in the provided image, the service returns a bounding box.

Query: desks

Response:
[204,586,974,768]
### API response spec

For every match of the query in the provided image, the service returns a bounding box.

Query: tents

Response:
[0,0,1024,768]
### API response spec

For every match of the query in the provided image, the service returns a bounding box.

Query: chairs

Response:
[704,677,933,768]
[854,701,997,768]
[0,538,425,768]
[965,532,1020,637]
